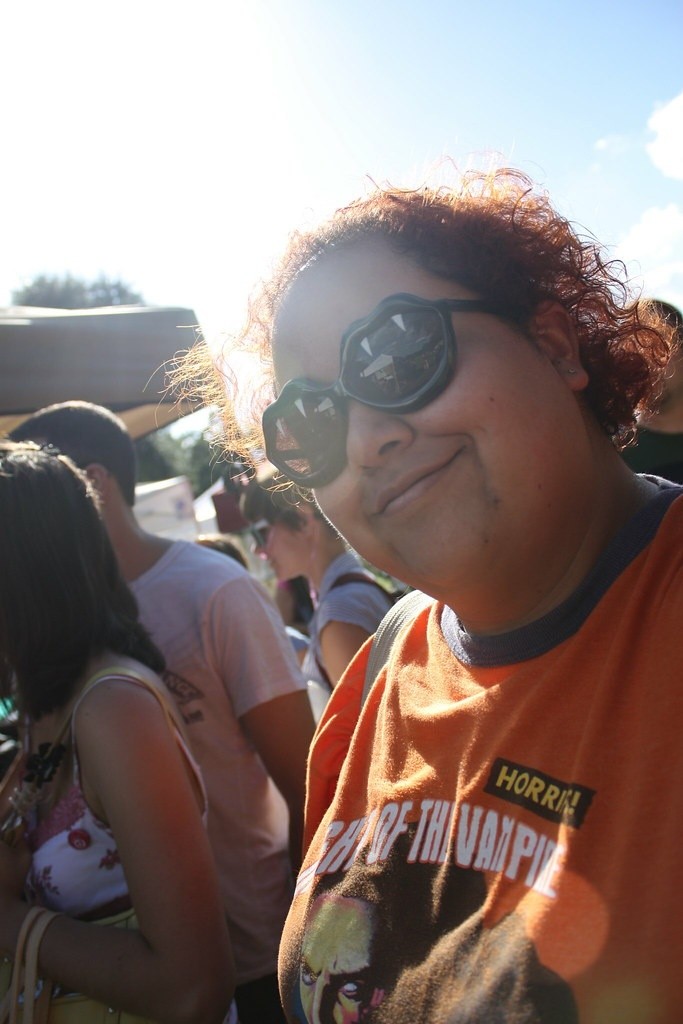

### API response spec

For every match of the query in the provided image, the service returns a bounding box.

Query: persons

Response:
[615,298,683,485]
[181,151,683,1024]
[0,403,405,1024]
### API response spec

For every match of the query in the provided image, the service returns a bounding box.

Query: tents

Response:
[0,305,245,448]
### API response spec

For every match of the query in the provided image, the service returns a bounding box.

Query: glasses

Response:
[250,518,272,548]
[262,292,498,487]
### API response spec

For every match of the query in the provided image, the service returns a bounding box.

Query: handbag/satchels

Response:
[1,909,150,1024]
